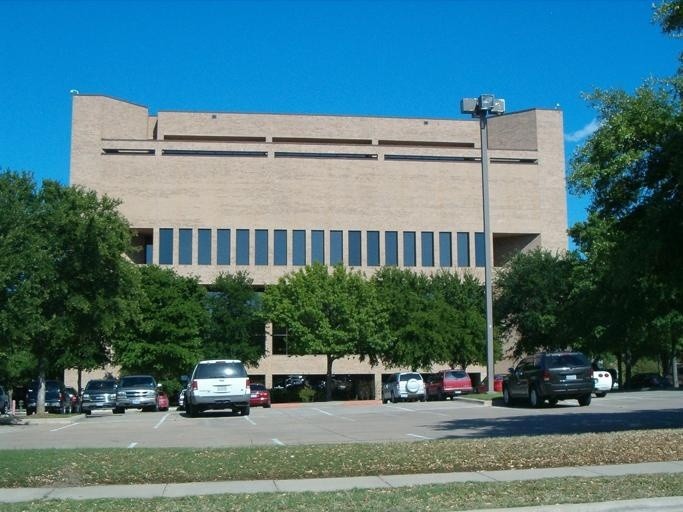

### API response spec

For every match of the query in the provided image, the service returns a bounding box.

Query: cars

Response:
[426,352,616,408]
[0,359,271,416]
[624,372,671,389]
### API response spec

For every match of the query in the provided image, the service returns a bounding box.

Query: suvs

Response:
[382,371,426,403]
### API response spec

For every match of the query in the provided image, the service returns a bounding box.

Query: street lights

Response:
[460,94,505,392]
